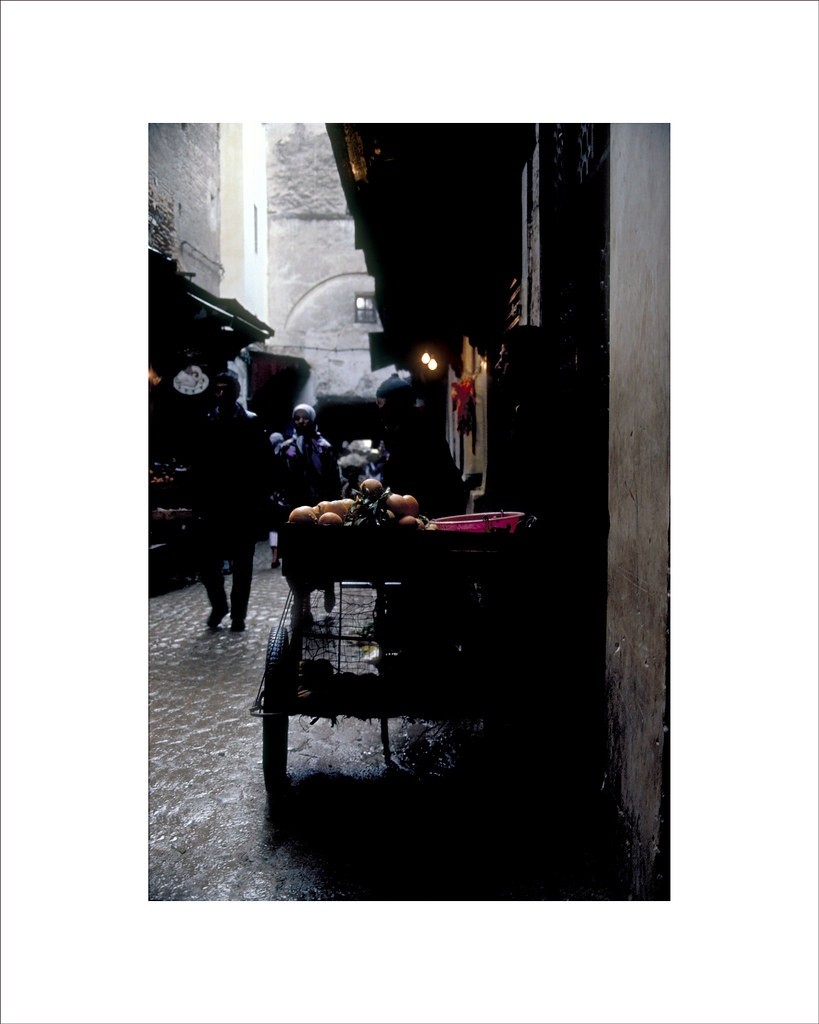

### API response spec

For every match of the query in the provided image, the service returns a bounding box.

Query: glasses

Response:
[213,386,232,394]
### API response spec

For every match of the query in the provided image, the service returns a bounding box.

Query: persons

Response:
[358,378,469,519]
[487,340,543,441]
[148,364,183,451]
[278,404,342,632]
[186,373,280,633]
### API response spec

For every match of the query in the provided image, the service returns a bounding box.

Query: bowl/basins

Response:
[430,512,525,533]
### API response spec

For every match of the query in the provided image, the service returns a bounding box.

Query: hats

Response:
[212,373,240,400]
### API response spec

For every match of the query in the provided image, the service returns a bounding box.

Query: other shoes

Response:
[324,591,335,614]
[230,617,245,632]
[271,558,280,569]
[206,602,230,628]
